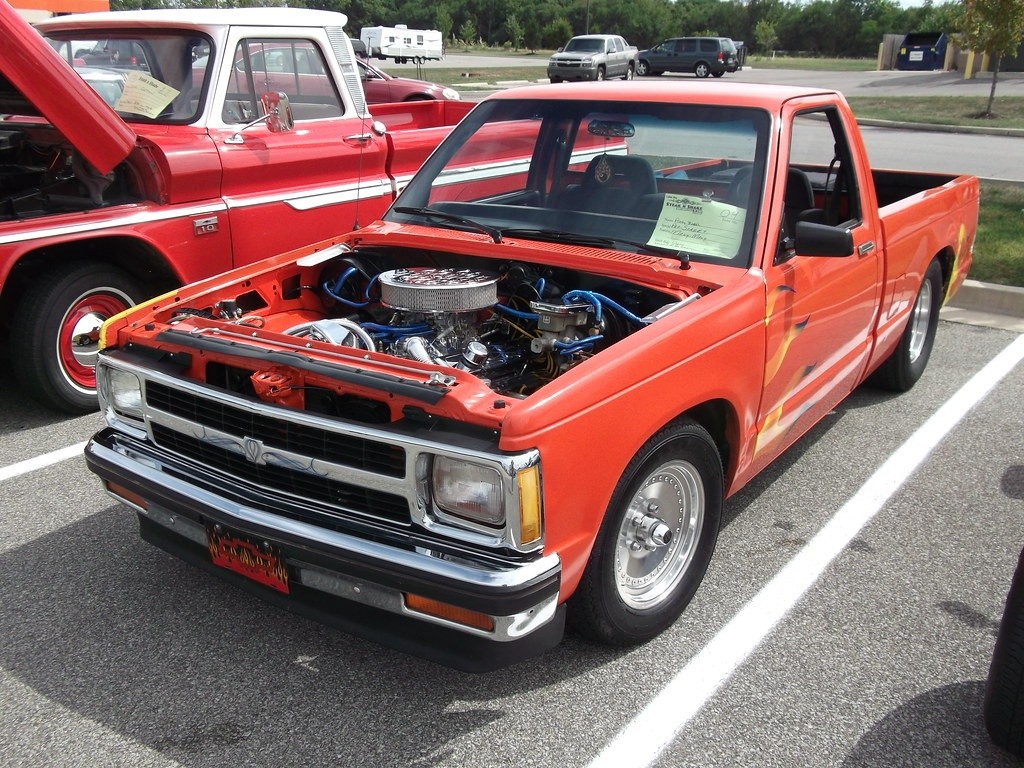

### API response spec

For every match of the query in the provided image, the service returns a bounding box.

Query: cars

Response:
[191,43,461,103]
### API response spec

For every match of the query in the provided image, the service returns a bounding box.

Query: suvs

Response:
[636,37,739,78]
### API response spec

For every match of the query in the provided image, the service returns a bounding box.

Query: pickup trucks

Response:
[547,34,640,83]
[1,1,628,416]
[84,82,981,675]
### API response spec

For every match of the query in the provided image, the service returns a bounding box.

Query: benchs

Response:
[184,101,340,125]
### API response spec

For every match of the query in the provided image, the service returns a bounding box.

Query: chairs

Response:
[726,163,818,240]
[557,152,657,217]
[298,54,322,75]
[636,193,722,221]
